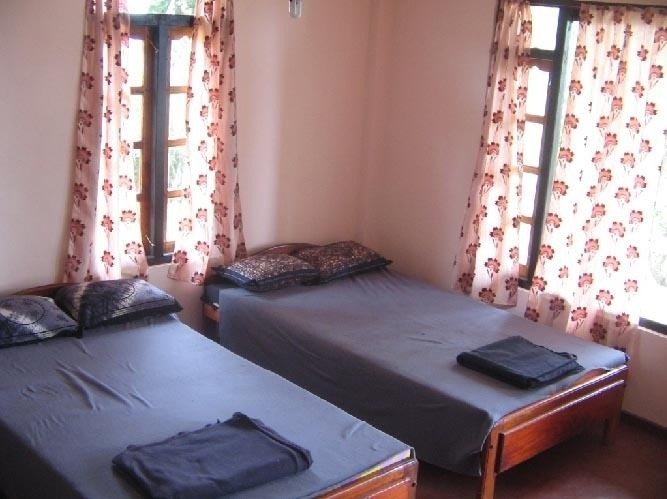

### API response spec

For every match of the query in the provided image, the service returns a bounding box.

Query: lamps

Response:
[288,0,302,18]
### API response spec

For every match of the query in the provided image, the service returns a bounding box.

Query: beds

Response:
[0,283,420,499]
[200,242,634,499]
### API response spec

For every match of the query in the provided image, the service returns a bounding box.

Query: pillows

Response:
[294,241,393,284]
[53,278,183,330]
[0,295,82,348]
[210,252,319,292]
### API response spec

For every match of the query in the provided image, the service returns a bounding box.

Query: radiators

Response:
[621,325,667,430]
